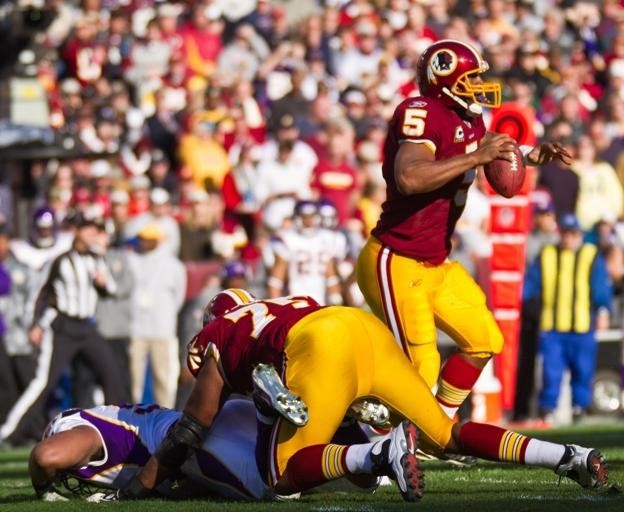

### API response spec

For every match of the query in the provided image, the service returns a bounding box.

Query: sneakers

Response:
[330,399,477,501]
[554,441,610,490]
[251,362,309,428]
[41,489,70,503]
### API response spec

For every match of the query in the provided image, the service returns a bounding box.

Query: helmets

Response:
[203,288,256,329]
[293,202,320,236]
[220,264,247,289]
[41,406,116,496]
[417,39,502,117]
[33,209,56,228]
[317,204,340,228]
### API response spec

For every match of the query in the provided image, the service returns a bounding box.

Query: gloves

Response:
[85,488,122,505]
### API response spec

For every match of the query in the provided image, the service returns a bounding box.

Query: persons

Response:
[28,362,391,503]
[1,1,623,303]
[522,212,613,430]
[82,286,622,502]
[94,245,137,402]
[1,215,31,447]
[356,39,573,467]
[9,206,72,438]
[0,220,119,450]
[115,224,187,408]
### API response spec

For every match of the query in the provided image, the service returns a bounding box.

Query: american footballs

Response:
[483,142,526,198]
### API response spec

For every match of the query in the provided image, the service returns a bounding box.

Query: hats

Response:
[137,223,164,239]
[559,214,580,232]
